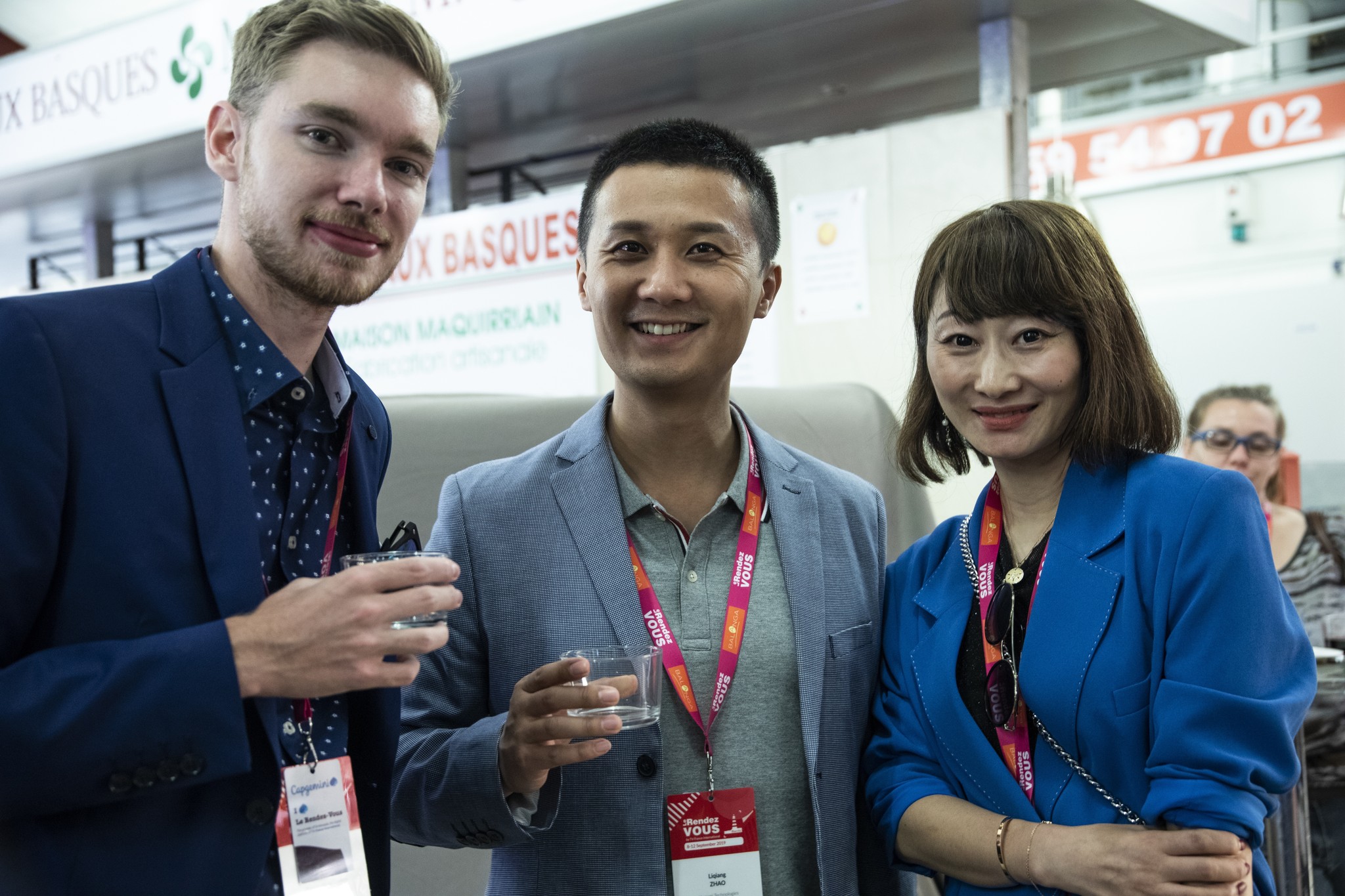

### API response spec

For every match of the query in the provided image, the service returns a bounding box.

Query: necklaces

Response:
[999,490,1059,587]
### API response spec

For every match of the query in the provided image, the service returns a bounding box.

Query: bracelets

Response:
[996,815,1052,896]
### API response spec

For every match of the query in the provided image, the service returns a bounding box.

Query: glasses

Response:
[1187,428,1281,457]
[984,577,1022,733]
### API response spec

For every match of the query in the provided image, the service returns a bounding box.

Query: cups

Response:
[559,647,658,731]
[339,547,450,629]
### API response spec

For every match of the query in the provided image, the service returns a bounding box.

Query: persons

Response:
[860,198,1317,896]
[0,0,465,896]
[384,116,919,896]
[1179,385,1345,896]
